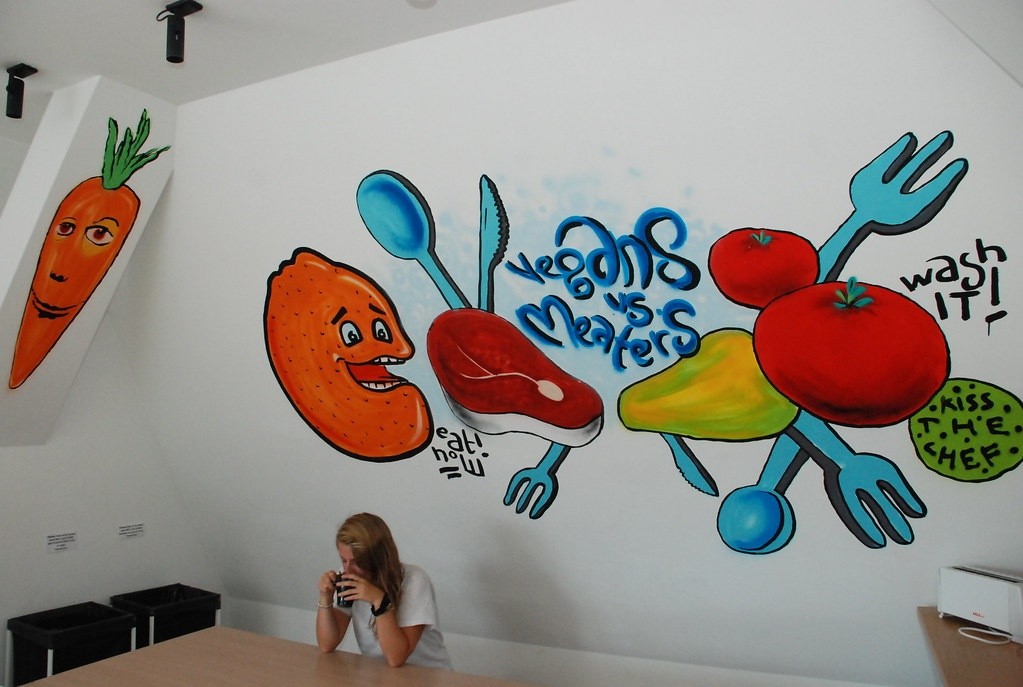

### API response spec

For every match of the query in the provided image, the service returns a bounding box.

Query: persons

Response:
[314,512,454,672]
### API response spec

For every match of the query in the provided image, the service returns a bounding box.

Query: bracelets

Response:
[318,600,334,608]
[370,592,392,616]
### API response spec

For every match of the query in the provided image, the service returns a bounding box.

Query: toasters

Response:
[937,563,1022,645]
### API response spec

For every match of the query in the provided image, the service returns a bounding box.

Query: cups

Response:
[335,575,355,607]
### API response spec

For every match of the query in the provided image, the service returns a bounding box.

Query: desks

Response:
[14,605,1023,687]
